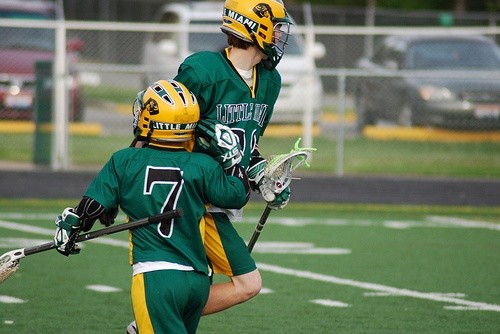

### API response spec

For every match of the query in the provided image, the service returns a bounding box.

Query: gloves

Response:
[247,158,291,211]
[196,118,244,170]
[53,207,81,256]
[99,207,115,227]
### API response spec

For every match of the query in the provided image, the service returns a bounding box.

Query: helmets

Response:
[132,79,200,152]
[220,0,295,71]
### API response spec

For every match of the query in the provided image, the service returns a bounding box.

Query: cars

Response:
[352,33,500,131]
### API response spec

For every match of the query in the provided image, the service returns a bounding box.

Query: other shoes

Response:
[126,321,138,334]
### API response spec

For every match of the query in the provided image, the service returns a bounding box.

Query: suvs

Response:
[139,4,326,123]
[0,0,86,122]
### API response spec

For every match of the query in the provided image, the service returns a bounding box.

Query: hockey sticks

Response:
[0,208,184,284]
[248,151,308,254]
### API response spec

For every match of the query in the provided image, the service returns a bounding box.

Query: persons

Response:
[125,0,294,334]
[53,79,251,334]
